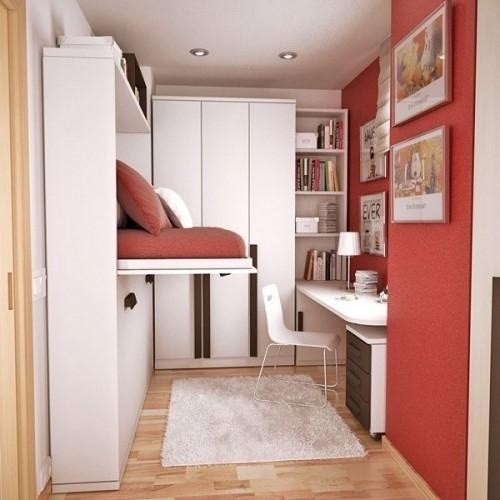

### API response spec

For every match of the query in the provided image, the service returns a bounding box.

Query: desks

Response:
[295,279,388,441]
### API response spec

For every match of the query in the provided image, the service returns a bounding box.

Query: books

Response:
[296,115,348,281]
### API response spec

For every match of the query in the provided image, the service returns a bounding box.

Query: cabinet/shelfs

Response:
[152,99,249,370]
[344,323,387,440]
[296,108,348,281]
[249,102,296,367]
[41,48,154,492]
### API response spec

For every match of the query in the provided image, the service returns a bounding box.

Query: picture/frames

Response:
[358,190,387,259]
[389,125,450,225]
[390,0,452,129]
[360,119,387,183]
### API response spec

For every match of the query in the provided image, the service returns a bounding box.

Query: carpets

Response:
[160,375,368,467]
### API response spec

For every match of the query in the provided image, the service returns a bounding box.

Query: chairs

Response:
[254,283,341,409]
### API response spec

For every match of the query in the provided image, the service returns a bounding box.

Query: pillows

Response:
[116,159,174,235]
[157,194,183,230]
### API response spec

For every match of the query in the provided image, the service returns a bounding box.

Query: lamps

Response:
[337,231,361,290]
